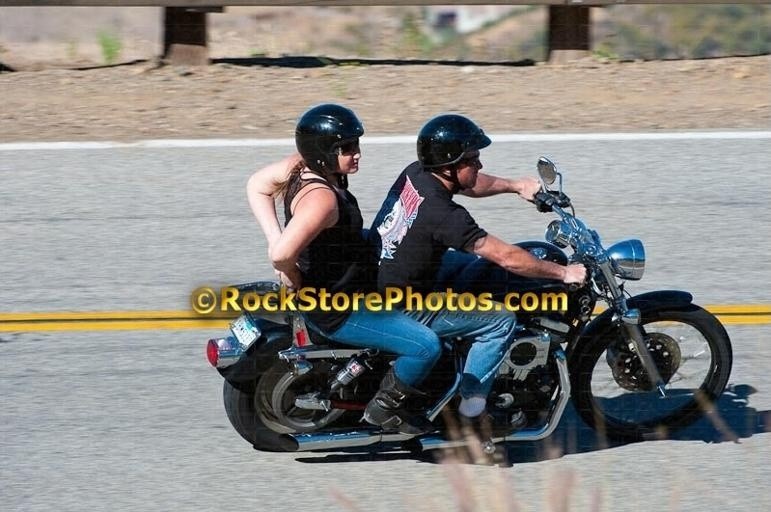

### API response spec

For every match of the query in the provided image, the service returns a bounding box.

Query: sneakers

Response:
[459,410,509,431]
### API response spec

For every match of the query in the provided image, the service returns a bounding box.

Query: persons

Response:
[368,115,587,464]
[247,104,442,436]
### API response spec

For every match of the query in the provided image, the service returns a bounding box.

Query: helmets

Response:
[417,115,491,172]
[296,104,364,173]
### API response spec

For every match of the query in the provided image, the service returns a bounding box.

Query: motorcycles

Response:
[207,157,732,453]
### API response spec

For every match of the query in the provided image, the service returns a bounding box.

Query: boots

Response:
[363,367,434,435]
